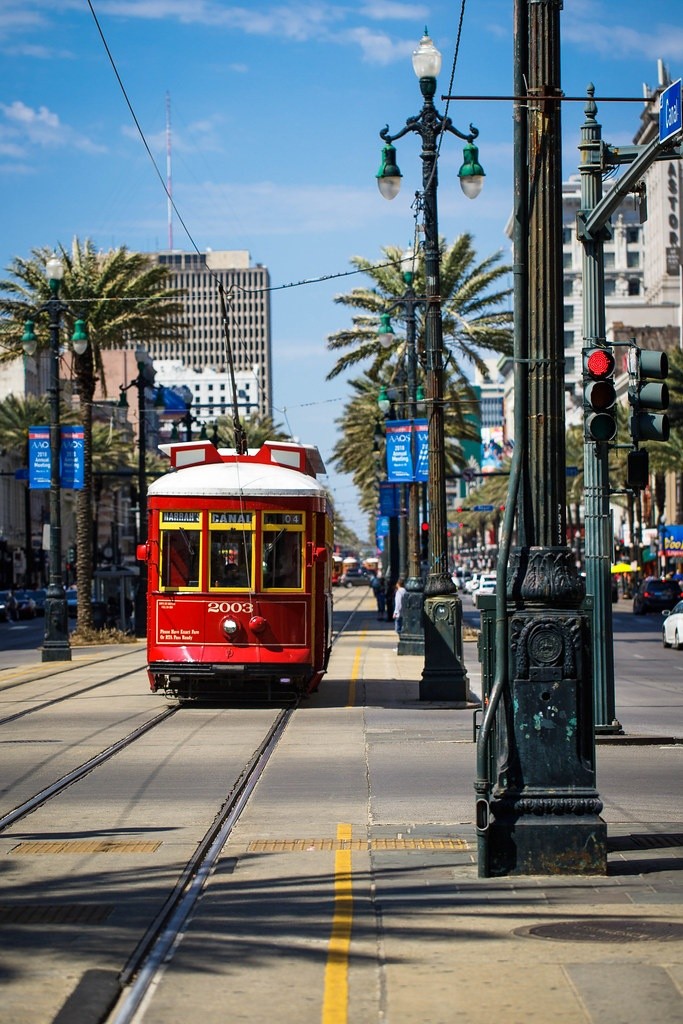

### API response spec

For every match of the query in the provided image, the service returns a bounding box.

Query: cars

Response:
[661,599,683,650]
[0,588,79,624]
[632,578,683,615]
[450,565,499,609]
[20,251,89,664]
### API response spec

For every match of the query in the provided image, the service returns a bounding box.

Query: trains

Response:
[135,438,336,706]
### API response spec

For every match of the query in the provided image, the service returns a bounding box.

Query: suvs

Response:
[340,572,371,589]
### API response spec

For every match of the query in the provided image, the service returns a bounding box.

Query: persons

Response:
[365,567,406,653]
[109,592,134,633]
[615,567,683,601]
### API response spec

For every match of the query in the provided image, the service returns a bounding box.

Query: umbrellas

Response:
[612,562,639,591]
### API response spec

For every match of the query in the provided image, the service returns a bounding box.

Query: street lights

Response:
[372,24,487,701]
[375,243,455,625]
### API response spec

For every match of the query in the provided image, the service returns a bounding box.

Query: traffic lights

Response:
[627,346,671,443]
[582,346,619,444]
[626,450,650,489]
[421,522,430,559]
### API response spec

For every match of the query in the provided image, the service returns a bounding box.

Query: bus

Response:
[113,344,212,637]
